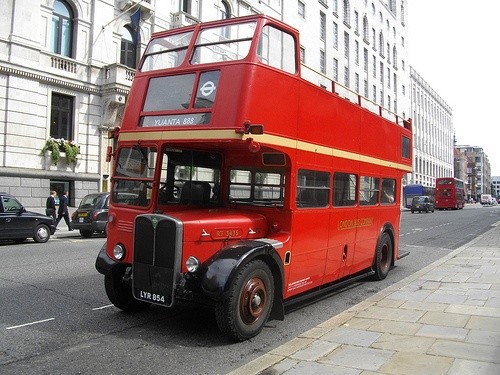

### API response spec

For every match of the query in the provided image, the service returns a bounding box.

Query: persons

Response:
[54,191,73,231]
[46,190,60,230]
[298,184,337,208]
[369,182,395,205]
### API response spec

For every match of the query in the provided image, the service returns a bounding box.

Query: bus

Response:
[405,184,435,209]
[94,13,413,343]
[435,177,465,210]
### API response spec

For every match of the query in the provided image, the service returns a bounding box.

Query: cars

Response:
[69,192,110,238]
[481,194,500,204]
[411,196,434,213]
[0,192,56,243]
